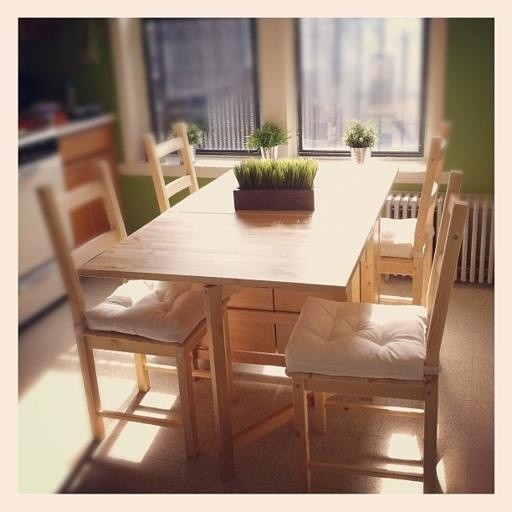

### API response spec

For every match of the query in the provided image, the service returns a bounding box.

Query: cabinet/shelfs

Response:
[58,124,125,248]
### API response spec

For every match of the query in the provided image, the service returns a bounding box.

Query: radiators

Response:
[384,193,495,286]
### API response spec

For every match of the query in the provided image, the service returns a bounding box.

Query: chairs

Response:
[142,120,199,382]
[377,127,451,306]
[284,169,469,493]
[35,159,238,458]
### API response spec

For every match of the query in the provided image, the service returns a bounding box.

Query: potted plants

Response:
[233,158,319,210]
[245,118,293,160]
[343,121,379,164]
[168,121,204,164]
